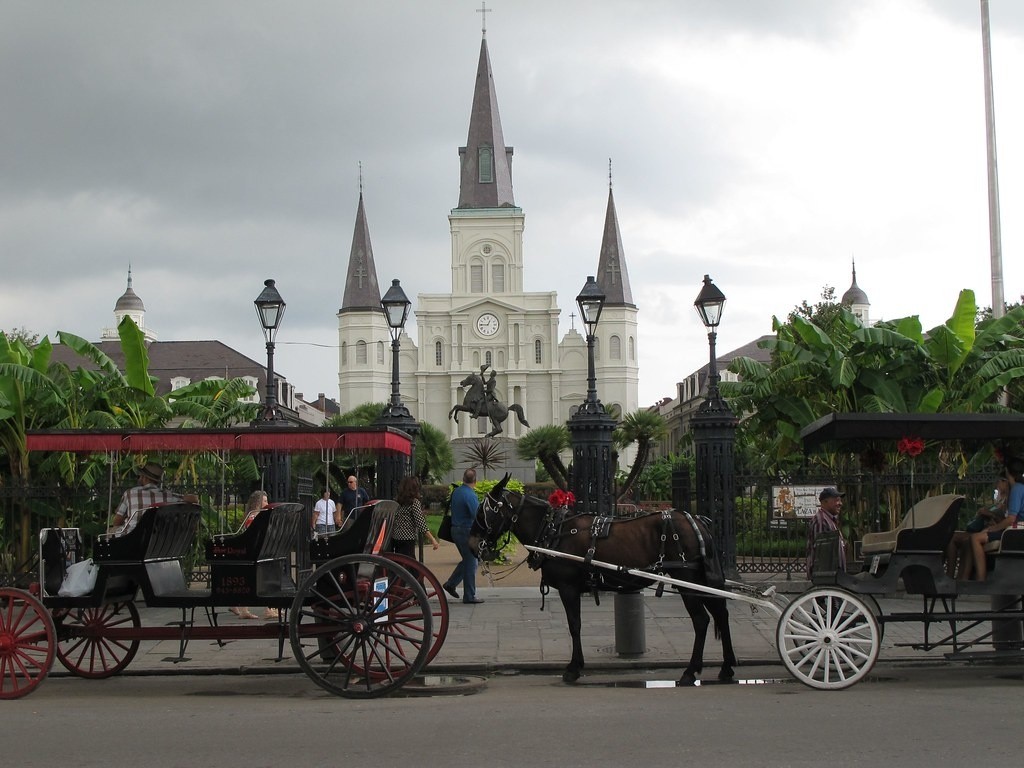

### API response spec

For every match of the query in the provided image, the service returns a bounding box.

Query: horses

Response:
[449,373,530,439]
[468,472,738,685]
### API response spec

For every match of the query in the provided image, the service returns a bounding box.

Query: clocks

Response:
[477,313,499,336]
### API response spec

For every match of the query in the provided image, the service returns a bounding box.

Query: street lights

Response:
[251,278,292,507]
[562,275,620,521]
[367,277,421,513]
[689,271,748,590]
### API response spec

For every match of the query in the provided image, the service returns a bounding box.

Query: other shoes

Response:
[229,607,241,614]
[443,583,460,598]
[264,609,286,618]
[464,598,484,604]
[238,610,258,620]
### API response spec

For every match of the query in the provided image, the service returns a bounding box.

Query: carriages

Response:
[466,411,1024,692]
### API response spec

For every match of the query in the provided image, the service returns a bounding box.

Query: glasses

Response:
[348,481,355,484]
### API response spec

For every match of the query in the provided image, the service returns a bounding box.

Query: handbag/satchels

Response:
[437,489,454,544]
[965,510,985,533]
[43,526,99,597]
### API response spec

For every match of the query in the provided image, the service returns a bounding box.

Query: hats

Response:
[998,471,1007,480]
[137,461,164,483]
[819,487,846,501]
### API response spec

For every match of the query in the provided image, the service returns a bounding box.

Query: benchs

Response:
[205,502,305,595]
[982,521,1024,554]
[309,500,400,591]
[861,494,967,551]
[90,501,203,597]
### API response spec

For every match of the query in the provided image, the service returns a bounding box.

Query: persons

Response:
[228,490,286,619]
[443,468,485,603]
[312,485,337,538]
[104,462,198,538]
[806,487,858,657]
[946,477,1010,578]
[391,476,439,606]
[958,454,1024,583]
[336,475,371,527]
[469,369,497,419]
